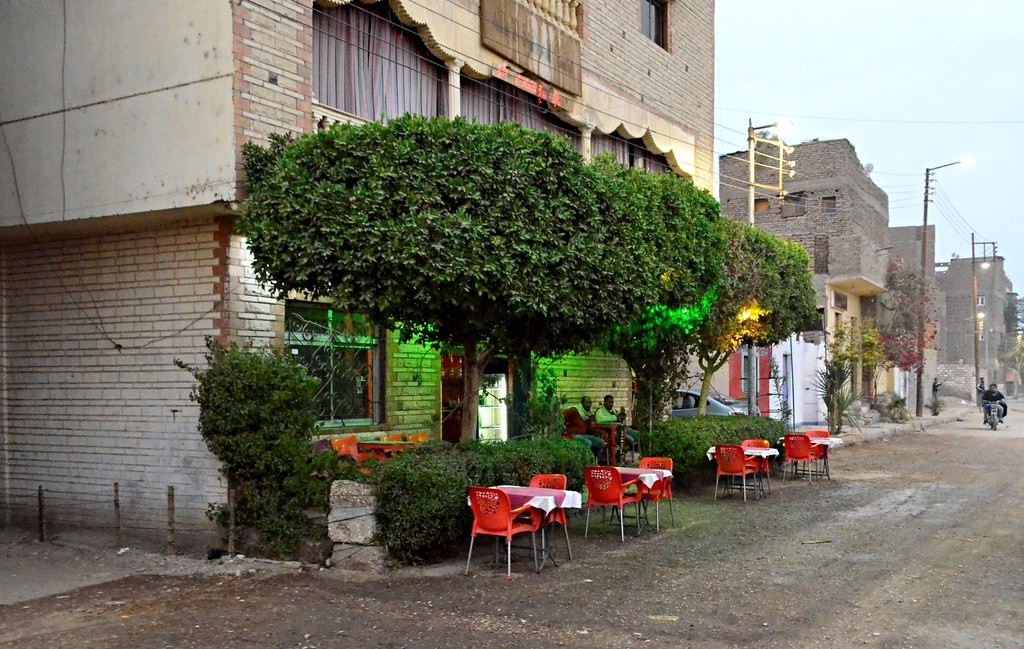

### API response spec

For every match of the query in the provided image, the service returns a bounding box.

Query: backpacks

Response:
[997,403,1007,417]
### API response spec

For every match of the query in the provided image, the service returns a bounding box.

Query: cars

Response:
[671,388,762,419]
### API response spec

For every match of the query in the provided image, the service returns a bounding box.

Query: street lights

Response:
[963,312,986,362]
[972,262,990,407]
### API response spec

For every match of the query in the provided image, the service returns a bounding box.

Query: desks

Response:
[706,447,779,500]
[491,485,581,574]
[615,468,673,538]
[357,441,415,457]
[777,436,843,480]
[590,423,625,466]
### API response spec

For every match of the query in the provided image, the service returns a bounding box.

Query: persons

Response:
[977,377,985,407]
[595,395,634,465]
[561,396,607,466]
[932,378,942,401]
[981,384,1006,424]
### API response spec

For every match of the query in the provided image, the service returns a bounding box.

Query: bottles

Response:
[442,357,462,377]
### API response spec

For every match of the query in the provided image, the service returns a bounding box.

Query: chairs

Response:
[682,395,691,408]
[782,431,831,484]
[313,432,428,466]
[625,425,642,463]
[465,474,574,580]
[714,439,771,503]
[584,458,678,542]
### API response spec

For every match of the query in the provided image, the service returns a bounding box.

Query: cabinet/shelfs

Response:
[478,373,507,445]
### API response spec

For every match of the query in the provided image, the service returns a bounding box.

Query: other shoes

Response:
[999,420,1003,423]
[984,421,988,424]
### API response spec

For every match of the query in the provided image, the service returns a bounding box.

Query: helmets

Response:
[984,403,991,409]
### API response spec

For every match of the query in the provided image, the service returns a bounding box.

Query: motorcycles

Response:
[986,400,999,431]
